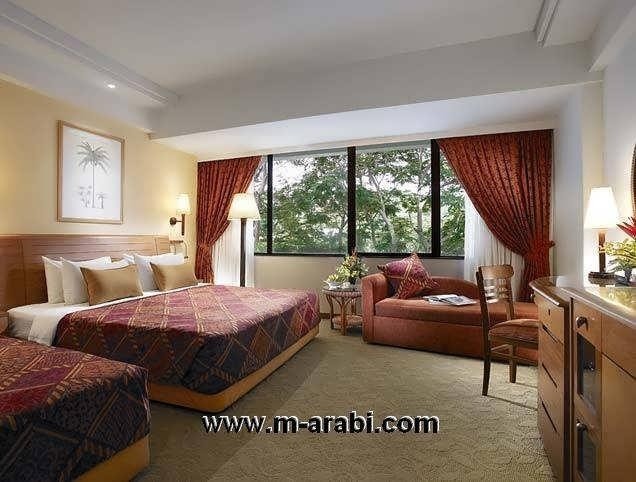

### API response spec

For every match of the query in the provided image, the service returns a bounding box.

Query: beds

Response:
[0,334,150,482]
[0,235,321,413]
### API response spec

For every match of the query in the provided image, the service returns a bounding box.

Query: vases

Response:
[623,268,632,281]
[348,277,356,291]
[328,283,339,290]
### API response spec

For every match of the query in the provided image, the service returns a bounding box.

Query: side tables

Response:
[322,285,362,336]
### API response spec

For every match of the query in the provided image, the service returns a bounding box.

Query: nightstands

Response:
[0,311,9,336]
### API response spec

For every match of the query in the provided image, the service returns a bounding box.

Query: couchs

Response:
[363,272,539,365]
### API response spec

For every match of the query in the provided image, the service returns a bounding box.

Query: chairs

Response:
[476,264,539,396]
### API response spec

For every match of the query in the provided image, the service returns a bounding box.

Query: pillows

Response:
[41,253,199,306]
[377,252,441,299]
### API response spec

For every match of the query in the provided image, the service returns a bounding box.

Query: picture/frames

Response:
[58,119,125,224]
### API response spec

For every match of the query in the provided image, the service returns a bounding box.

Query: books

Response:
[423,293,477,306]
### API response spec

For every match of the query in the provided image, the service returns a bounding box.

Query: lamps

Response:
[228,193,261,287]
[584,186,620,279]
[170,194,190,235]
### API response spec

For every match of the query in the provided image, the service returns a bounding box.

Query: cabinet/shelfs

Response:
[566,294,636,481]
[533,289,566,482]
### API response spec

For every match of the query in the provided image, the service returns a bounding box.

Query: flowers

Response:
[597,217,636,273]
[323,272,345,283]
[335,251,370,279]
